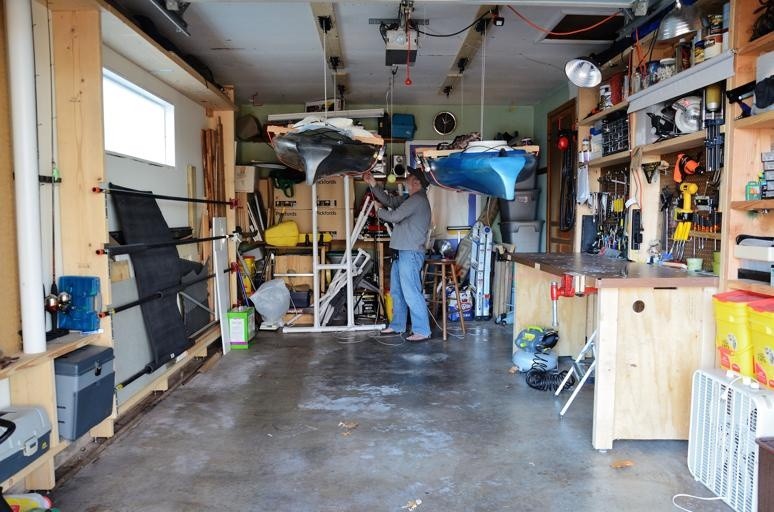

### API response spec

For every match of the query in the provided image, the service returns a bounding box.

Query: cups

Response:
[686,257,704,272]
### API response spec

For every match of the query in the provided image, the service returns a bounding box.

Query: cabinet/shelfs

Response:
[0,331,118,493]
[576,0,774,297]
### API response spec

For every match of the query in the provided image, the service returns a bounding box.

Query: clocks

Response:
[432,111,457,136]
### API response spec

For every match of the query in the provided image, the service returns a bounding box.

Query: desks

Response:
[509,252,719,453]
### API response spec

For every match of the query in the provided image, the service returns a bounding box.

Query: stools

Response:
[422,259,466,342]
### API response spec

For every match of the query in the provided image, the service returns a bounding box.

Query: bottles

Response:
[582,139,591,152]
[746,181,761,201]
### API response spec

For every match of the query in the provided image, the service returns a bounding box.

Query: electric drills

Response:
[673,154,705,183]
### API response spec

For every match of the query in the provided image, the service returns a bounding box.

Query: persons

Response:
[363,166,433,342]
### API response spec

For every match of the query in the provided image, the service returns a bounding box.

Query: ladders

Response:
[468,221,491,321]
[555,329,598,416]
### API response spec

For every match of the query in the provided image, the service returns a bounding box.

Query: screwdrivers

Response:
[668,221,691,261]
[693,212,717,257]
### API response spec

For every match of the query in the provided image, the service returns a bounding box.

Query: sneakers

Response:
[407,333,432,341]
[381,324,396,334]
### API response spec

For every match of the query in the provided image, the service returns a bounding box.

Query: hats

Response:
[407,166,429,188]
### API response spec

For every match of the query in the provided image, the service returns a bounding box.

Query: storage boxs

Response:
[234,166,356,327]
[54,345,116,440]
[226,307,256,349]
[498,156,545,254]
[0,403,53,482]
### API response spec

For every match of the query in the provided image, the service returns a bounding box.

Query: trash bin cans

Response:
[755,437,774,512]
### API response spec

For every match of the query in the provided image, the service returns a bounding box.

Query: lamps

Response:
[564,52,603,88]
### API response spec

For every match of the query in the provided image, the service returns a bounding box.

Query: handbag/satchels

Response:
[271,169,305,199]
